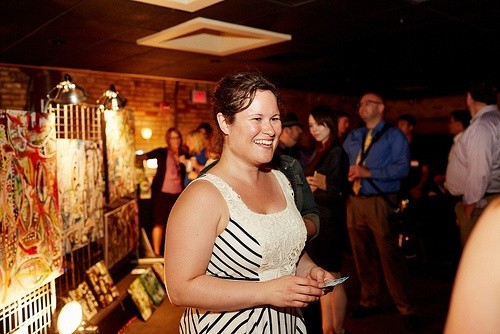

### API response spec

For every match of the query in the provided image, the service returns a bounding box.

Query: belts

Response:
[460,193,496,201]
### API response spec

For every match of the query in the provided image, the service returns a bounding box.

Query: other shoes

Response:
[343,250,353,260]
[352,306,385,318]
[407,316,422,332]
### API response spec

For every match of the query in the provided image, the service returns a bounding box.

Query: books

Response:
[138,267,166,305]
[127,278,156,321]
[85,260,120,309]
[151,262,164,283]
[67,280,101,322]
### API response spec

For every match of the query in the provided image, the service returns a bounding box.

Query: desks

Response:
[116,294,187,334]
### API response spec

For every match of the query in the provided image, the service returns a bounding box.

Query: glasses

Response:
[357,100,381,107]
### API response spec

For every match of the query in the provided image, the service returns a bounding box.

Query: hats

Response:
[280,112,302,128]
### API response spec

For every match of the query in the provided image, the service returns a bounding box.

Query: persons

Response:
[273,102,351,334]
[163,71,336,334]
[135,121,218,257]
[445,77,500,252]
[342,92,413,317]
[397,109,472,282]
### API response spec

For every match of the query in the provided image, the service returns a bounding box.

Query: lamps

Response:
[44,73,129,114]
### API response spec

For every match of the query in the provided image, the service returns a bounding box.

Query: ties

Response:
[352,128,373,196]
